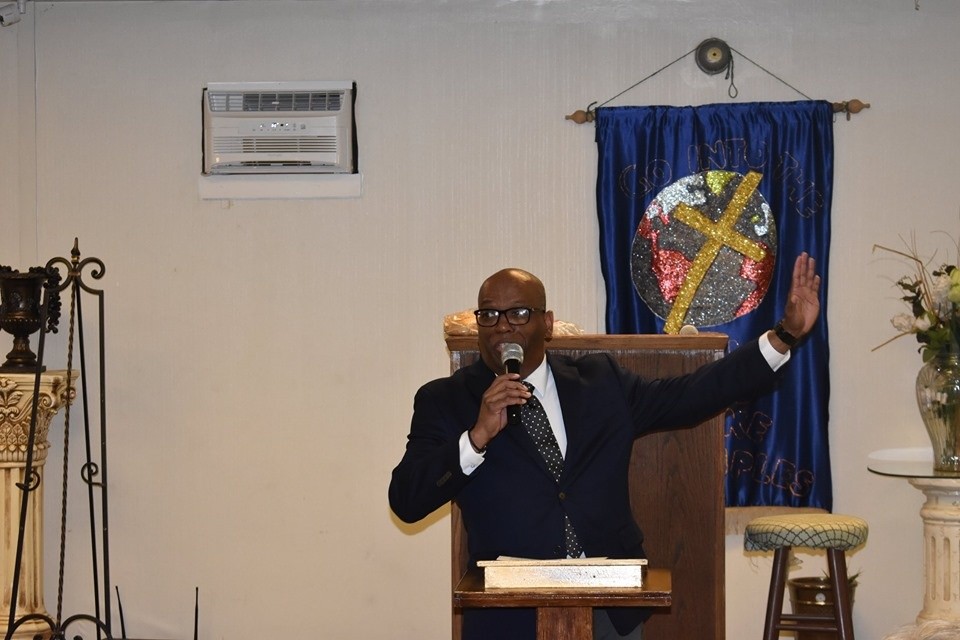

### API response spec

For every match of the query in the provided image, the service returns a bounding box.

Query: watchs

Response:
[773,319,799,346]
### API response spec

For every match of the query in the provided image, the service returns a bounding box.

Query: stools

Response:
[744,513,867,640]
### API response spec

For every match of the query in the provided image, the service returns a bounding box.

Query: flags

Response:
[590,99,835,536]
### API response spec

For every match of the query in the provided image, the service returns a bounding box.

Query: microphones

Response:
[500,342,524,425]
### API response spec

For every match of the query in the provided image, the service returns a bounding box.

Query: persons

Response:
[386,251,822,639]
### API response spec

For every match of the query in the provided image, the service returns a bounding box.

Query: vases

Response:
[918,352,960,472]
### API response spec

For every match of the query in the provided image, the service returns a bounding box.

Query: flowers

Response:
[869,228,960,362]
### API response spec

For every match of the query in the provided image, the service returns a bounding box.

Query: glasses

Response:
[474,307,545,327]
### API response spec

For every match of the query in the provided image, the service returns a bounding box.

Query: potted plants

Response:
[788,570,861,640]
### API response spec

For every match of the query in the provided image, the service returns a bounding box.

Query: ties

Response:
[521,382,583,559]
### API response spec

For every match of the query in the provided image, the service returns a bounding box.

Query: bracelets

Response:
[469,427,488,453]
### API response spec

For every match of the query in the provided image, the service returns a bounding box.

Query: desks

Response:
[866,449,960,640]
[452,564,672,640]
